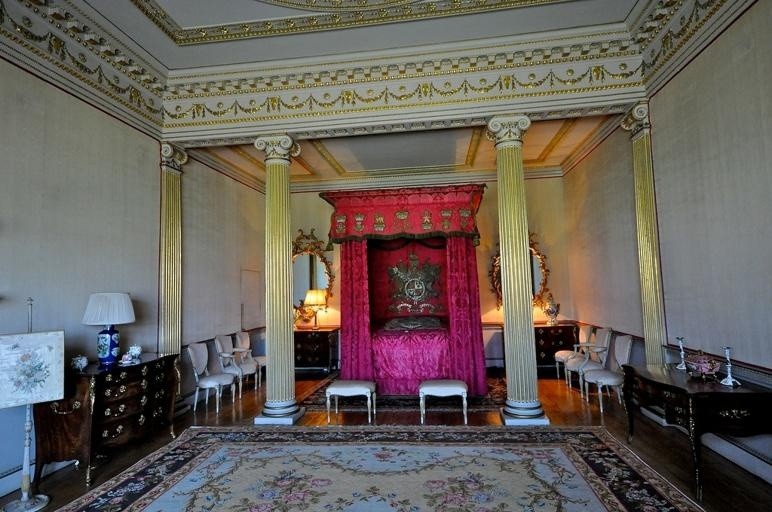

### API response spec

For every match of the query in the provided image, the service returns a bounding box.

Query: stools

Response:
[326,379,377,425]
[419,380,469,424]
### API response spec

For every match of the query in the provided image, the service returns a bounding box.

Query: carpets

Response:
[56,423,707,512]
[296,371,505,411]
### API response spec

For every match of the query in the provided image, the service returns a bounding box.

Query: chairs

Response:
[554,325,634,413]
[187,331,264,414]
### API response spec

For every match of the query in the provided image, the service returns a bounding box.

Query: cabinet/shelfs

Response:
[294,328,338,379]
[534,324,576,377]
[29,350,179,488]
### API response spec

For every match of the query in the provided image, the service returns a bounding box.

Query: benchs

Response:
[640,405,771,512]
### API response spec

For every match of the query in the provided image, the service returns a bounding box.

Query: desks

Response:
[620,363,772,503]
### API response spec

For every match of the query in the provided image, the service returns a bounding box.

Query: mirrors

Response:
[292,227,335,325]
[490,233,549,310]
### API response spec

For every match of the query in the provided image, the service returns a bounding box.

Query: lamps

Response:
[81,292,139,368]
[303,290,327,329]
